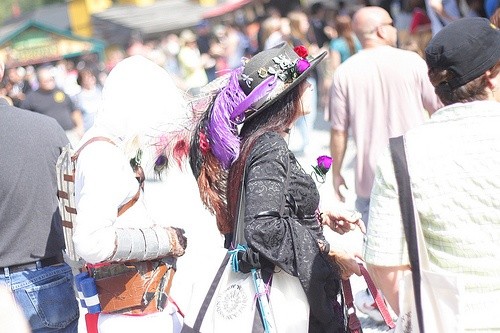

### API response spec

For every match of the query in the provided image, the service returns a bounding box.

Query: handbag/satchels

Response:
[182,243,310,333]
[341,261,399,333]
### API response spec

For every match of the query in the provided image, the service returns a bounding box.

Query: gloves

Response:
[164,226,187,257]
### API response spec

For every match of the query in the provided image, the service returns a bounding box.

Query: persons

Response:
[0,0,500,333]
[363,17,500,333]
[152,41,366,333]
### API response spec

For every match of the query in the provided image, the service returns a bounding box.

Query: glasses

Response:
[370,22,394,33]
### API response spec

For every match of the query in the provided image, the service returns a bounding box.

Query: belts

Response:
[0,257,64,275]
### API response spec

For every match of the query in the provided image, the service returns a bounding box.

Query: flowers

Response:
[311,154,334,183]
[294,46,308,58]
[294,58,310,75]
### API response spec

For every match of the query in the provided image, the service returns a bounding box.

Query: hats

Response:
[425,17,500,93]
[150,42,327,235]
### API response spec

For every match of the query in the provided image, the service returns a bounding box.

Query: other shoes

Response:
[355,290,390,323]
